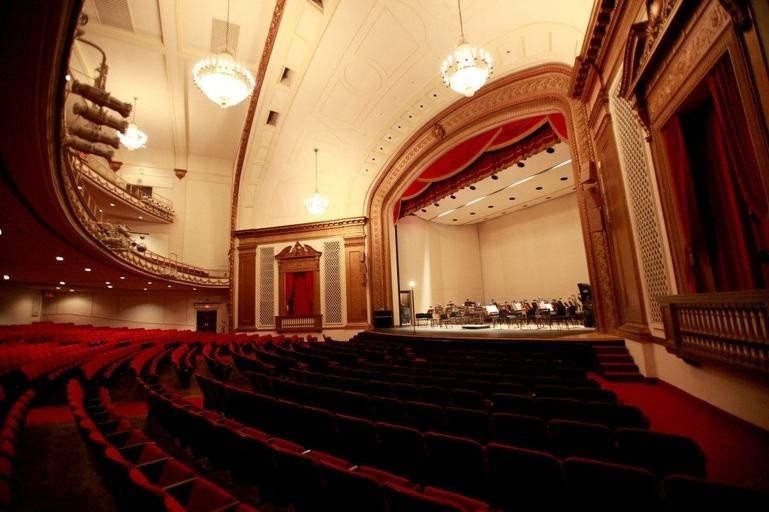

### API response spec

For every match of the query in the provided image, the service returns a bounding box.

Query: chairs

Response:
[3,324,761,512]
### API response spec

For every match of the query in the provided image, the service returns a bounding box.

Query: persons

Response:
[425,296,579,327]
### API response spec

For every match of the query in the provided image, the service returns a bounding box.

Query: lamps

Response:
[66,36,132,165]
[438,1,495,97]
[117,96,148,151]
[191,2,257,110]
